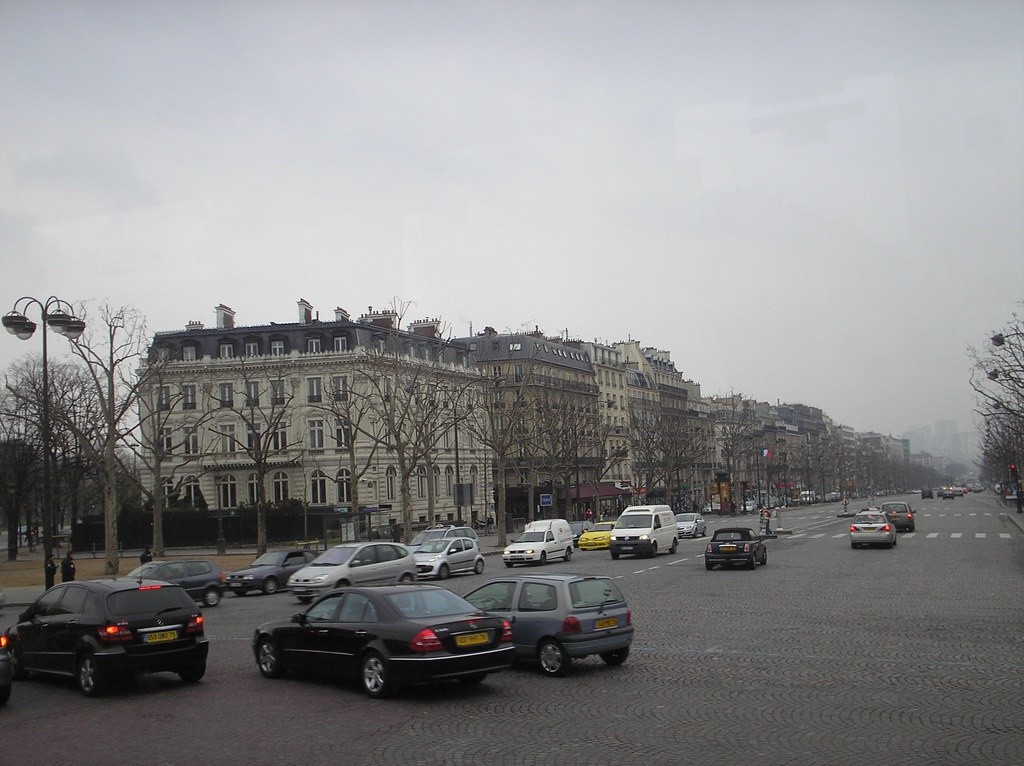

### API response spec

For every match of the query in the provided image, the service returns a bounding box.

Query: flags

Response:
[760,448,773,457]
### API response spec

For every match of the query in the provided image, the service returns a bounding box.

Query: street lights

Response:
[403,376,506,527]
[0,295,88,564]
[649,425,703,513]
[534,398,616,521]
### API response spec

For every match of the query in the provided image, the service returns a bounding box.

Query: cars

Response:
[461,571,635,678]
[224,548,321,596]
[876,501,917,532]
[701,481,986,514]
[413,536,485,580]
[252,580,517,699]
[568,520,594,548]
[674,513,707,539]
[285,540,418,603]
[850,508,898,550]
[703,527,768,571]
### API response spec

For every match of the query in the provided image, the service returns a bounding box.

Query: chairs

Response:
[352,603,374,622]
[174,564,207,577]
[395,596,414,614]
[540,587,555,609]
[518,590,531,607]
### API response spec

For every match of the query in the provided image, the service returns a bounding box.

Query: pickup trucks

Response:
[501,517,575,568]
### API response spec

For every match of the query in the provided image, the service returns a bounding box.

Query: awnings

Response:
[558,484,630,501]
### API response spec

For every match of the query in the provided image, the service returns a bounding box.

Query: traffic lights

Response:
[1009,462,1016,477]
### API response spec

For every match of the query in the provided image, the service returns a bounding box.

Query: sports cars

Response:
[577,520,617,551]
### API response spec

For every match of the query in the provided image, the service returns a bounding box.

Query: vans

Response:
[609,504,680,560]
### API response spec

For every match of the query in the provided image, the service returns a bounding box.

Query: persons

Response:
[45,552,59,592]
[585,508,593,521]
[729,502,736,517]
[487,515,494,535]
[60,552,76,583]
[140,546,152,564]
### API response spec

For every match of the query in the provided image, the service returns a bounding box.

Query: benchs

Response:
[92,541,123,558]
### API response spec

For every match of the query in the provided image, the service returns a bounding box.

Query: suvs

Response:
[406,523,481,552]
[2,577,210,698]
[122,559,226,610]
